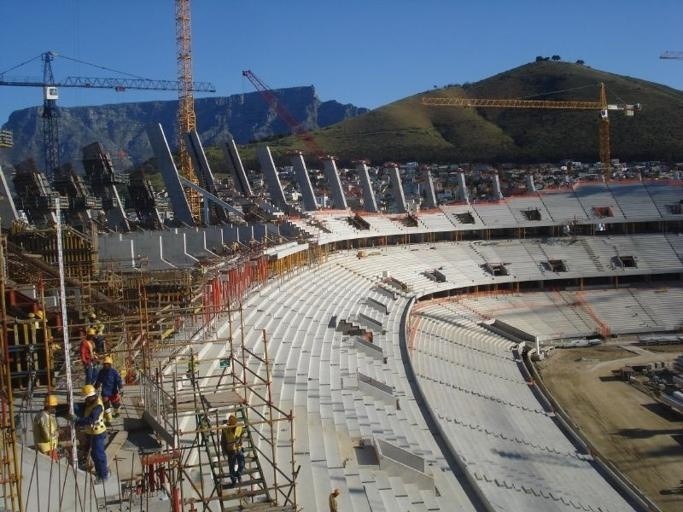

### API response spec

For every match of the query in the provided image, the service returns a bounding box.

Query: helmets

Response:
[101,356,113,365]
[86,313,96,335]
[81,383,96,396]
[44,395,59,407]
[35,311,43,318]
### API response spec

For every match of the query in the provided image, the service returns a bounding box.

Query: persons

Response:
[93,354,124,426]
[198,412,211,447]
[71,382,108,481]
[185,352,199,385]
[31,392,66,462]
[328,488,340,512]
[78,326,98,389]
[219,413,245,485]
